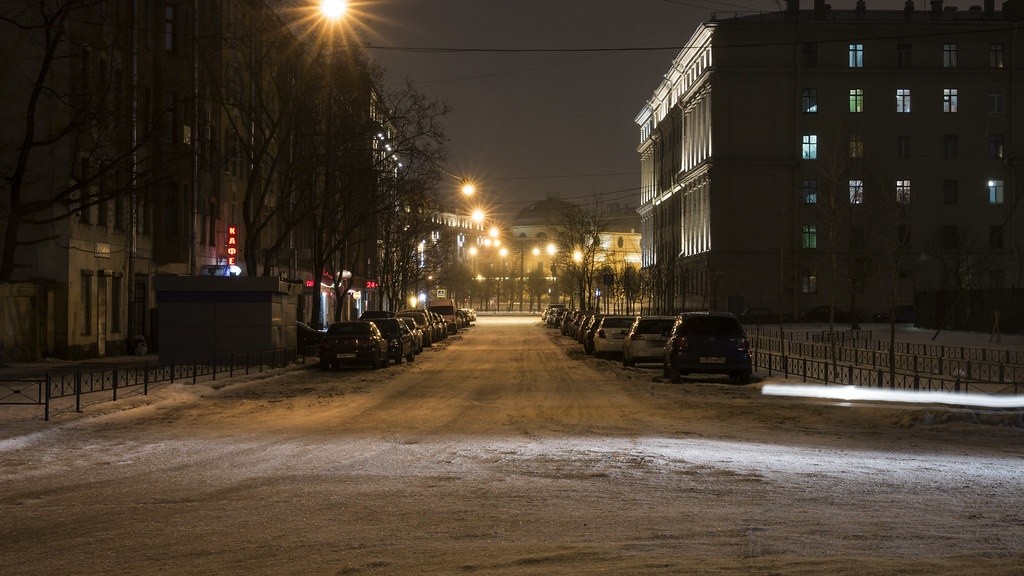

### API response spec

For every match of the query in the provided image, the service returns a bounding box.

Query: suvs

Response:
[664,312,753,384]
[595,315,636,357]
[623,316,681,365]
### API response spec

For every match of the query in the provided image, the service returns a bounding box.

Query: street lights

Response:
[416,159,474,305]
[548,244,557,286]
[484,225,500,310]
[500,249,509,302]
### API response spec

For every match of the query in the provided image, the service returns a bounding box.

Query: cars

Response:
[297,321,326,349]
[398,310,447,347]
[543,304,603,354]
[363,318,417,365]
[321,322,388,370]
[358,311,398,326]
[401,318,424,354]
